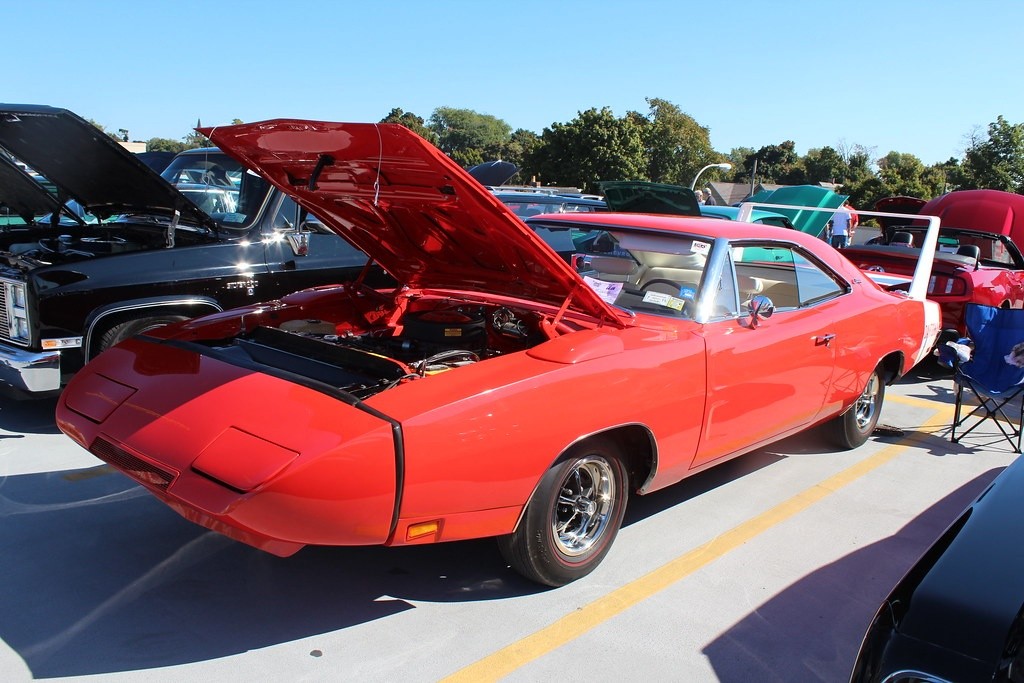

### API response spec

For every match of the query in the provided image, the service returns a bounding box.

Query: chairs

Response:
[737,274,764,313]
[590,257,638,293]
[957,245,981,261]
[937,303,1024,453]
[891,231,913,244]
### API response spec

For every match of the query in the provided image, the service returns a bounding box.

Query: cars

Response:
[848,454,1023,682]
[864,196,928,246]
[466,159,634,273]
[53,119,941,590]
[834,189,1024,348]
[0,103,397,402]
[573,180,851,262]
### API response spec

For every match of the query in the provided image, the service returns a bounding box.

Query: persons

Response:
[702,188,716,205]
[695,190,705,205]
[825,201,858,248]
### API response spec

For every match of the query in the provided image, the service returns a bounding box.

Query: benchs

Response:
[640,266,799,310]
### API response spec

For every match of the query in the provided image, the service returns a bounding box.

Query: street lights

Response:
[690,163,732,190]
